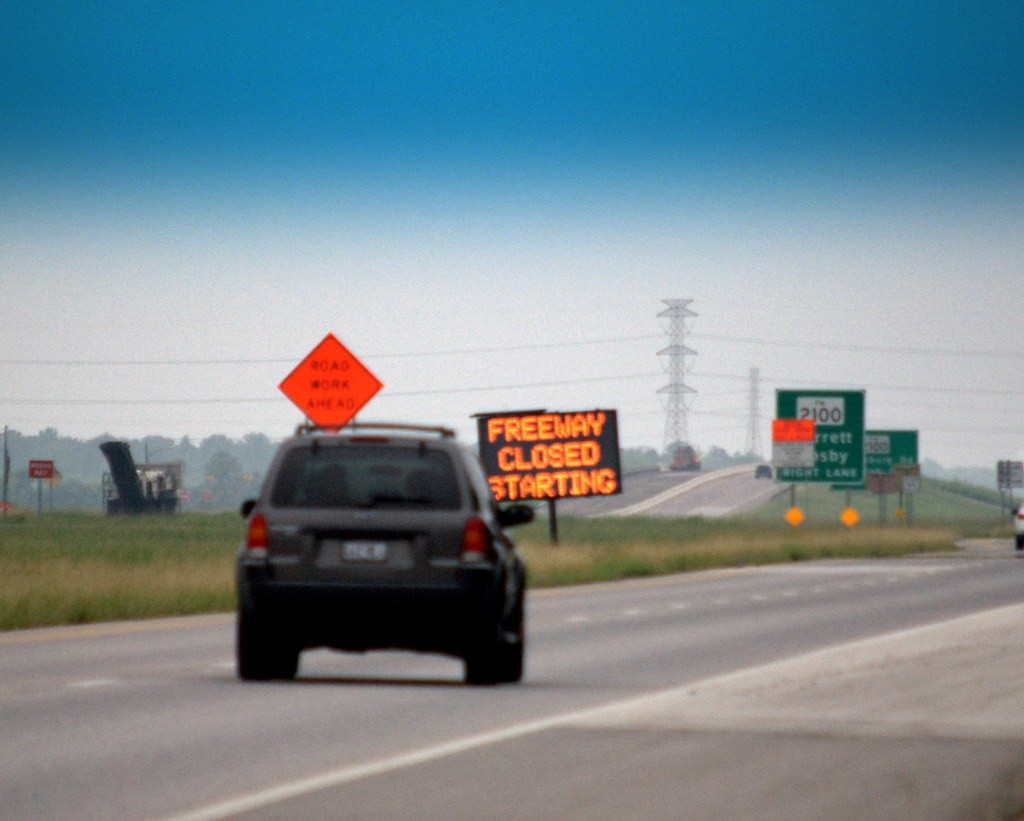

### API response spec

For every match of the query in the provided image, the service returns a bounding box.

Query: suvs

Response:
[235,420,537,686]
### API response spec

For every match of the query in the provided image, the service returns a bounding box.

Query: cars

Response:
[756,465,773,479]
[1010,502,1024,550]
